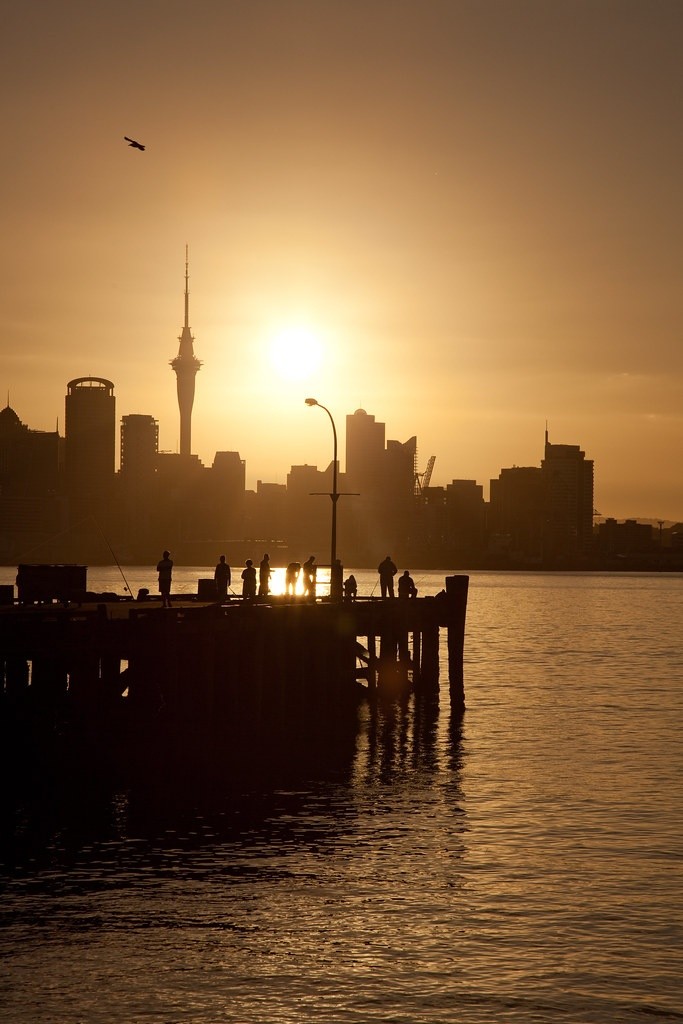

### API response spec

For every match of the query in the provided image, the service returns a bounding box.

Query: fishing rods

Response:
[92,514,135,600]
[370,575,381,598]
[413,574,431,587]
[210,566,240,600]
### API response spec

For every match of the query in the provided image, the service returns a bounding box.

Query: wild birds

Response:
[123,136,145,151]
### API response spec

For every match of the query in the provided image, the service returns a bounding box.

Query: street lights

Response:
[305,398,362,599]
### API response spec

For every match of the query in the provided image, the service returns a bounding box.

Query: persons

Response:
[258,554,275,596]
[156,550,174,607]
[378,556,398,598]
[215,556,231,603]
[334,559,343,604]
[241,558,257,606]
[344,575,357,603]
[399,570,418,600]
[285,555,318,596]
